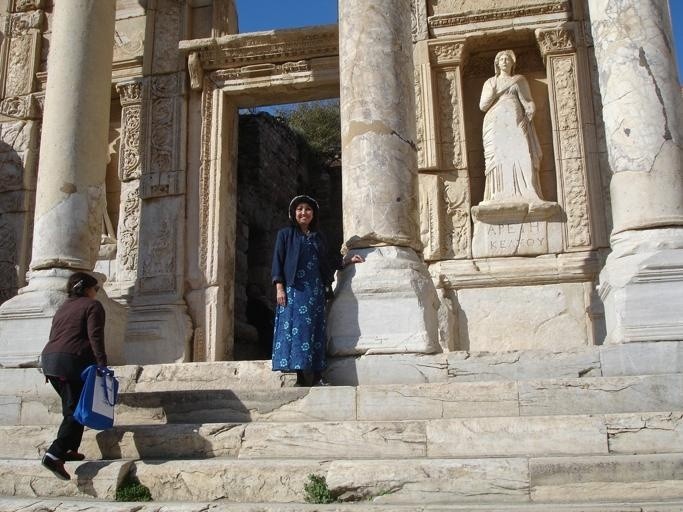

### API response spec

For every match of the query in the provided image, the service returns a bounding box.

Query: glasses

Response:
[93,285,100,292]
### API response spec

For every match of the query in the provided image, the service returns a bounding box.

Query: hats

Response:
[288,195,320,224]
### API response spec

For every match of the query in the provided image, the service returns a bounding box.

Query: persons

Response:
[271,195,363,386]
[40,272,107,481]
[479,49,543,206]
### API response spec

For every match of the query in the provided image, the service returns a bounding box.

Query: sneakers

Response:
[312,377,332,386]
[42,455,70,480]
[294,377,308,387]
[65,450,85,461]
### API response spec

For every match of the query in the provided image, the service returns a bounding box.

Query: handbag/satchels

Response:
[73,365,119,430]
